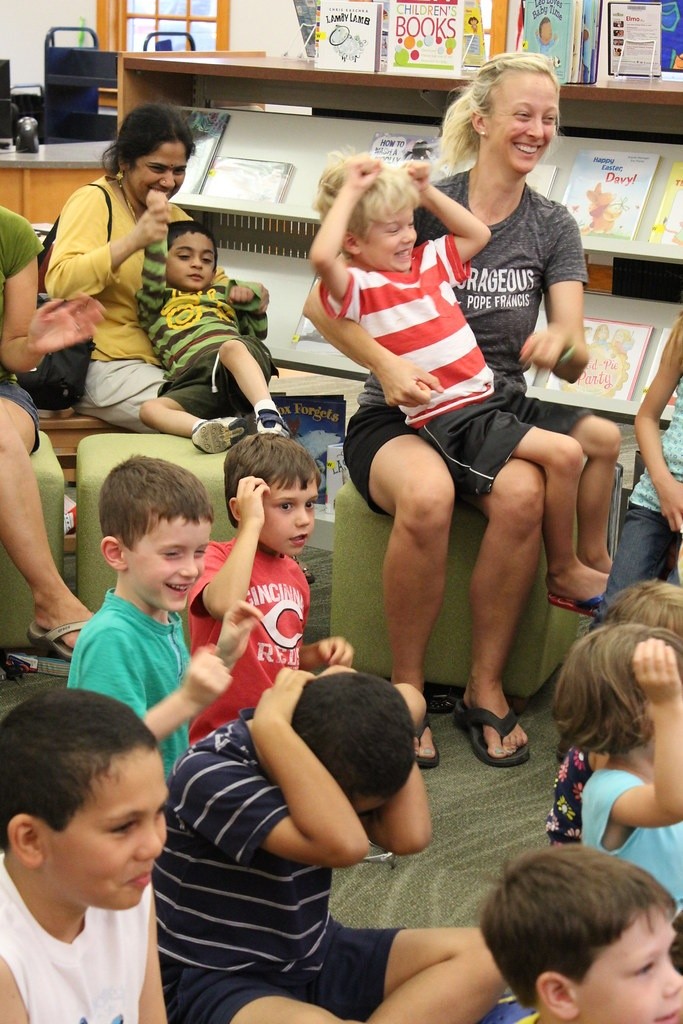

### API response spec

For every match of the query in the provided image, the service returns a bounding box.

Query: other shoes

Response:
[557,737,571,761]
[479,992,539,1024]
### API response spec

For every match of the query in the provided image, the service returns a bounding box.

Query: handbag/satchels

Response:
[14,184,112,410]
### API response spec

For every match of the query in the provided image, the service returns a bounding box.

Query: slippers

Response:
[547,590,607,617]
[26,612,95,663]
[414,713,439,767]
[452,699,529,767]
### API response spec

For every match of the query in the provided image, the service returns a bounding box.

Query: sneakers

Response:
[191,417,248,454]
[256,409,290,439]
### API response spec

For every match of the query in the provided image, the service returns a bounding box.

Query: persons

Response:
[340,48,591,767]
[478,847,683,1024]
[306,152,622,617]
[185,427,354,747]
[0,689,175,1024]
[67,457,266,786]
[545,579,683,979]
[132,187,289,455]
[150,664,509,1024]
[44,104,233,438]
[0,204,100,659]
[590,315,683,639]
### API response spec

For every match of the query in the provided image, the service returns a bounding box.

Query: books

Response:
[314,0,683,87]
[559,145,683,247]
[365,129,559,201]
[544,316,681,406]
[293,269,350,355]
[167,105,231,196]
[199,155,295,204]
